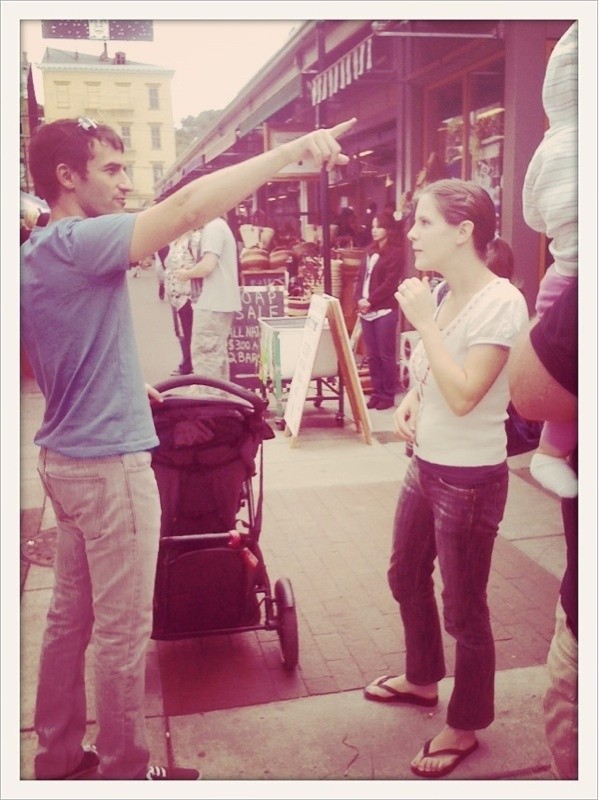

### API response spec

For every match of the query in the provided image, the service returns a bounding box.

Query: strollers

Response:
[147,374,298,671]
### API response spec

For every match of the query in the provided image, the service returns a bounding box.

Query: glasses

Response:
[49,117,99,158]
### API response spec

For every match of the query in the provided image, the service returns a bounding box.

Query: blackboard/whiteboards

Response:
[284,292,374,438]
[227,284,289,382]
[239,270,289,288]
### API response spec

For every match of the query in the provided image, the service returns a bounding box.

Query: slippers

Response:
[409,730,479,777]
[363,675,438,707]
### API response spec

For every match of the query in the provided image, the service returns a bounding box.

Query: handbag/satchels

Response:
[500,398,544,456]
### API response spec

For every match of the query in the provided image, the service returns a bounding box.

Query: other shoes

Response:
[367,396,380,408]
[376,400,395,410]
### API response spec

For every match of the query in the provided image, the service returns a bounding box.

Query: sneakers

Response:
[58,743,100,780]
[137,765,201,780]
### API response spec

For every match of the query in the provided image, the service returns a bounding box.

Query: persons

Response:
[353,210,407,410]
[20,117,358,780]
[363,178,531,778]
[126,227,203,376]
[506,20,578,781]
[173,214,243,381]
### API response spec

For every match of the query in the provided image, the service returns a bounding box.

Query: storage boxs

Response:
[258,317,339,380]
